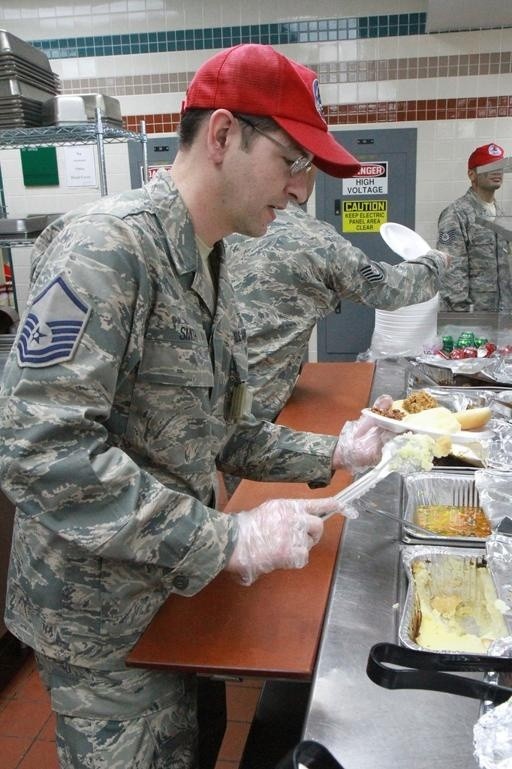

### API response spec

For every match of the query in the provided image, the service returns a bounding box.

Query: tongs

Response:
[311,431,425,522]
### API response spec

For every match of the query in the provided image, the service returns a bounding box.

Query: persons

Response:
[1,42,406,769]
[436,142,512,313]
[221,200,450,497]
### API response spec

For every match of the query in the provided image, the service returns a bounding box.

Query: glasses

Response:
[215,109,314,178]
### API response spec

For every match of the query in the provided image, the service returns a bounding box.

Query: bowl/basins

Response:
[402,471,512,541]
[0,27,60,128]
[43,93,124,123]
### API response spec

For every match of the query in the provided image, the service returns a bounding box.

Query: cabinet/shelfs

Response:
[0,106,150,380]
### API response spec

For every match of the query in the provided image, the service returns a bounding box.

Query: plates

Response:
[378,220,431,262]
[362,400,497,442]
[368,292,439,361]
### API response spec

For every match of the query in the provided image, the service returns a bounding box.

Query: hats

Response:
[180,40,362,188]
[463,141,512,174]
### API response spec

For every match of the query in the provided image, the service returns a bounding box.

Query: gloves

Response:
[334,412,408,478]
[233,496,362,587]
